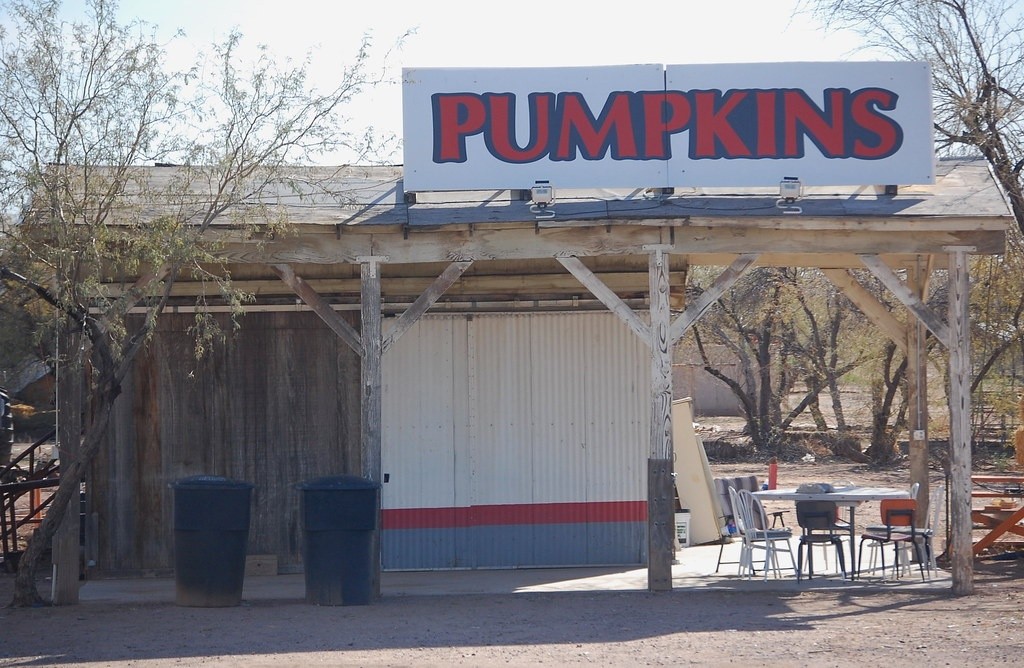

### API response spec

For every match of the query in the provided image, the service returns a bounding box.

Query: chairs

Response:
[714,475,944,585]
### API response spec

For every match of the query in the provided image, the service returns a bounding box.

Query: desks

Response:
[752,485,910,583]
[0,475,85,575]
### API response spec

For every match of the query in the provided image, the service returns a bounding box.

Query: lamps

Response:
[530,178,556,219]
[776,176,804,214]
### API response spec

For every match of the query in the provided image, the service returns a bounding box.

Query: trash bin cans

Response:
[298,473,384,606]
[170,474,253,609]
